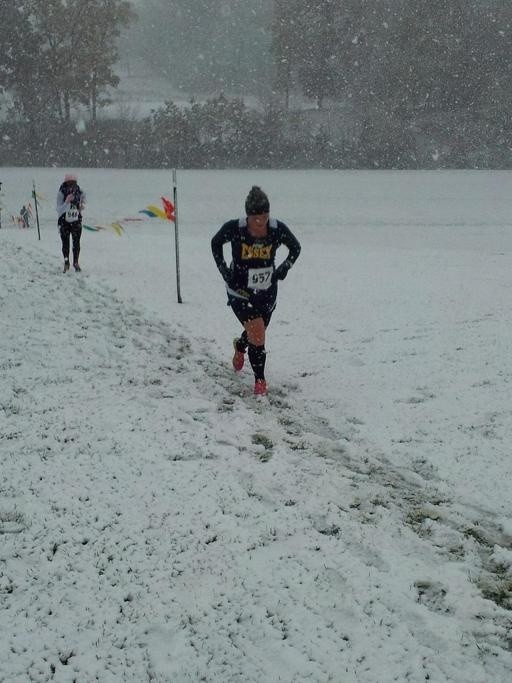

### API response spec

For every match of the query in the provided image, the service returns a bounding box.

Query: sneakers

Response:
[73,263,81,272]
[232,337,244,371]
[63,263,70,273]
[253,378,266,397]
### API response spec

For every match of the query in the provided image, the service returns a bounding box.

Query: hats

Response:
[63,173,78,182]
[245,185,270,215]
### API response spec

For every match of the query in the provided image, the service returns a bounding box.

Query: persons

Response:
[56,172,87,272]
[210,184,301,395]
[20,204,31,227]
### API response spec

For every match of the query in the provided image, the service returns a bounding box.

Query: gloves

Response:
[217,261,233,283]
[272,260,292,281]
